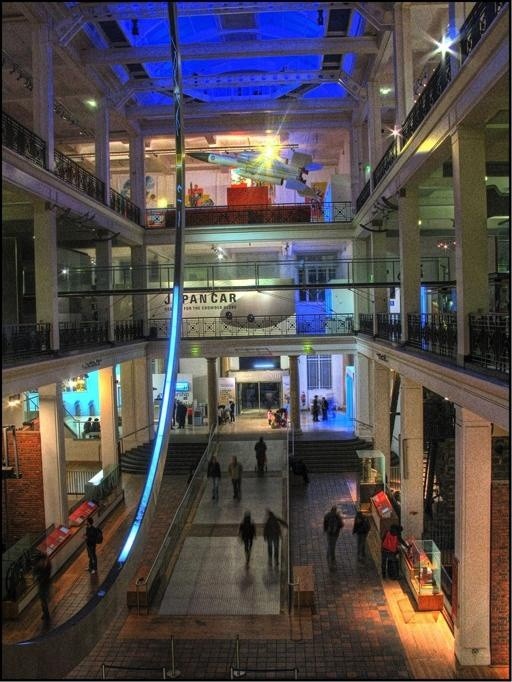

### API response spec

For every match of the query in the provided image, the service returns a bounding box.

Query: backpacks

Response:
[382,528,398,553]
[91,527,103,544]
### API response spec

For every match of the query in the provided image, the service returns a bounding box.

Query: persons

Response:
[352,512,371,556]
[86,517,97,575]
[33,552,51,619]
[254,437,267,474]
[207,456,220,499]
[237,511,256,561]
[312,395,328,421]
[381,524,412,580]
[84,417,101,432]
[218,402,234,425]
[323,506,344,567]
[264,512,288,566]
[171,400,187,429]
[269,410,288,429]
[228,456,243,500]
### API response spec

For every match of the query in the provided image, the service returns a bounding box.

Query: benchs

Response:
[126,565,160,607]
[291,564,316,608]
